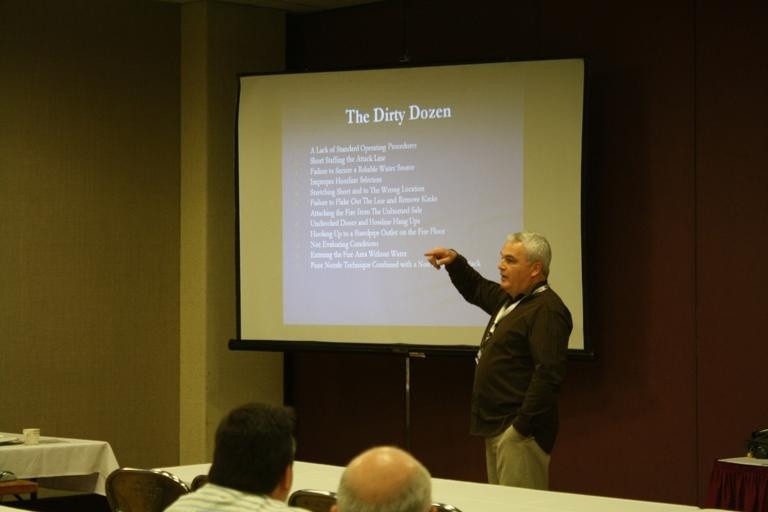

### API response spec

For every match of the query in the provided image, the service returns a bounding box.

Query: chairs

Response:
[103,466,337,512]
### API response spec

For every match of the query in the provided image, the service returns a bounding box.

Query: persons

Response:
[421,230,573,490]
[161,401,314,512]
[329,444,437,512]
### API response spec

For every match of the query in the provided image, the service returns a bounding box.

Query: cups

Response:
[22,428,41,446]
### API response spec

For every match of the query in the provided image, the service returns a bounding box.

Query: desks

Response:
[122,454,701,512]
[1,431,116,511]
